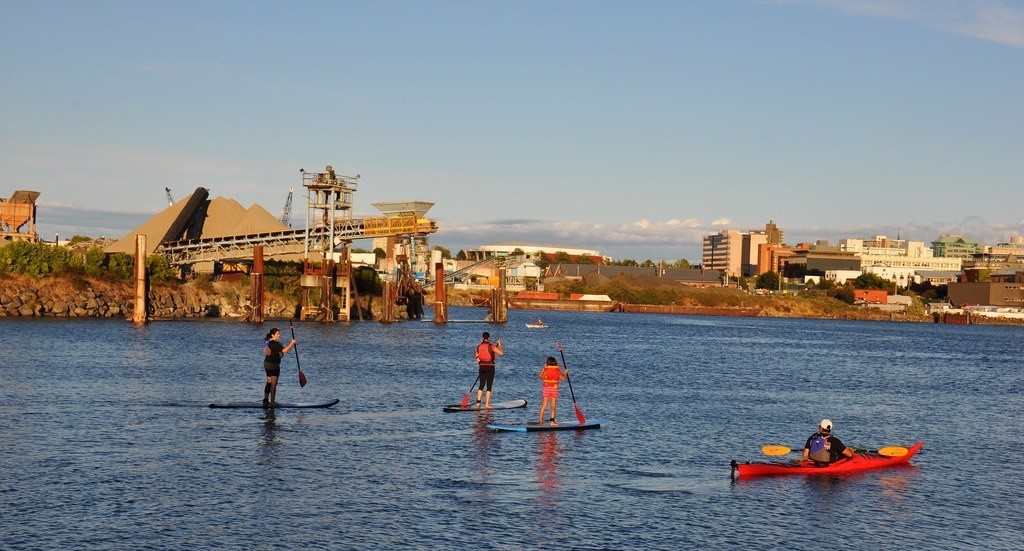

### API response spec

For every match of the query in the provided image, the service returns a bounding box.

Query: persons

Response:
[538,356,568,425]
[803,419,853,466]
[474,332,504,408]
[263,327,298,407]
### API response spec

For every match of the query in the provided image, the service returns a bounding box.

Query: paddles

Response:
[558,341,585,424]
[761,445,908,457]
[288,316,307,387]
[459,342,500,408]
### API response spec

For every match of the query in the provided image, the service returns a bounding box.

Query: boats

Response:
[730,442,922,482]
[525,323,549,328]
[509,292,622,312]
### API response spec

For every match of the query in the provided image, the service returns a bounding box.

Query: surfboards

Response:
[443,399,528,412]
[209,398,340,408]
[484,419,609,431]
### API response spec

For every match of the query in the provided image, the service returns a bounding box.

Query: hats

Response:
[817,418,833,430]
